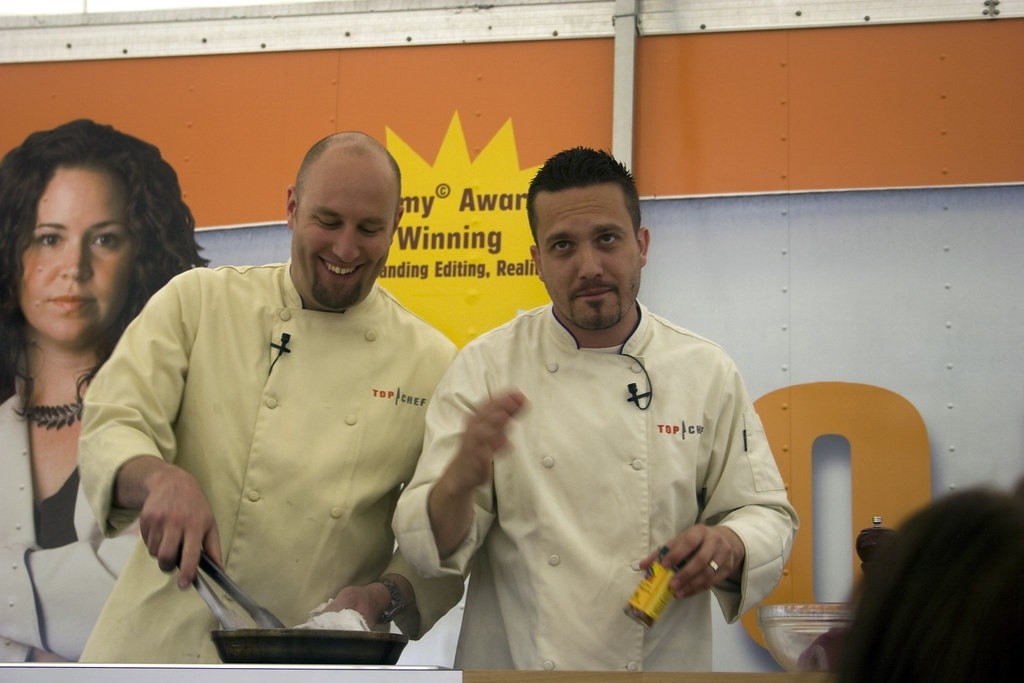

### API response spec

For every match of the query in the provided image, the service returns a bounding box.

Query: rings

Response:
[709,560,718,572]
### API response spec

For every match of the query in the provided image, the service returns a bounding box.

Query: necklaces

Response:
[27,401,83,431]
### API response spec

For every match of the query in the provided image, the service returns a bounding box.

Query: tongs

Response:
[172,543,289,631]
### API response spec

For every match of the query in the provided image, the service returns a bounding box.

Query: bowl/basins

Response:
[754,604,858,673]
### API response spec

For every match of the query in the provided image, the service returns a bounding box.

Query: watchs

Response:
[371,578,404,625]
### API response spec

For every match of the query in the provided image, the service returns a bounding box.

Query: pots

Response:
[215,624,409,664]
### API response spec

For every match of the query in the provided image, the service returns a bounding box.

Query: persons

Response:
[0,120,209,662]
[390,147,798,674]
[79,131,458,664]
[840,491,1024,682]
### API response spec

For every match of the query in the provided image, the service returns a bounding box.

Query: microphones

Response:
[278,333,291,355]
[628,383,640,408]
